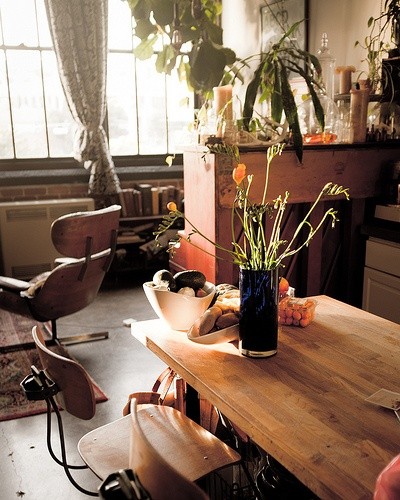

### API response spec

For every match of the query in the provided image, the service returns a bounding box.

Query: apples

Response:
[278,277,289,295]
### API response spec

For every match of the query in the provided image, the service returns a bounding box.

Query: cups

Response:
[221,120,240,145]
[239,117,258,145]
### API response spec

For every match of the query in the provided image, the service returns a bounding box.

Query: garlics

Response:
[153,285,168,290]
[176,287,196,297]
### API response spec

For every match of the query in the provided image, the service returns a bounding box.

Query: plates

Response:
[364,389,400,411]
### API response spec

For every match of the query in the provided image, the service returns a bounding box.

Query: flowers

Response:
[152,136,349,271]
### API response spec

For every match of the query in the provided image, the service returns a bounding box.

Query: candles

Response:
[338,67,351,94]
[349,82,369,142]
[213,84,232,138]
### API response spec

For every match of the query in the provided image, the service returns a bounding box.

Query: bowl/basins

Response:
[187,306,239,346]
[143,281,216,332]
[276,297,320,328]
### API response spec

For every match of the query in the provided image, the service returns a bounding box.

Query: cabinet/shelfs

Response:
[168,139,400,296]
[362,234,400,325]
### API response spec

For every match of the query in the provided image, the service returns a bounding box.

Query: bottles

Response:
[331,100,350,144]
[310,33,336,135]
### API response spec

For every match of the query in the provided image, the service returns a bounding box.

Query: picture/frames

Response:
[259,0,309,77]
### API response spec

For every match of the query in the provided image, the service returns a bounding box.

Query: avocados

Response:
[152,269,206,290]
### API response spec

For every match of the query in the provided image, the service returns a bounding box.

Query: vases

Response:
[238,265,278,358]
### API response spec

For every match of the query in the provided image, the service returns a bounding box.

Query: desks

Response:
[131,295,400,500]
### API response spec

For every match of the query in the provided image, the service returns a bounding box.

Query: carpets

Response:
[0,310,107,422]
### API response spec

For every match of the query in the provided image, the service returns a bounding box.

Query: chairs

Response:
[19,325,241,500]
[0,204,122,354]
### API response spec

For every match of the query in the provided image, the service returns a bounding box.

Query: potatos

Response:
[197,306,240,336]
